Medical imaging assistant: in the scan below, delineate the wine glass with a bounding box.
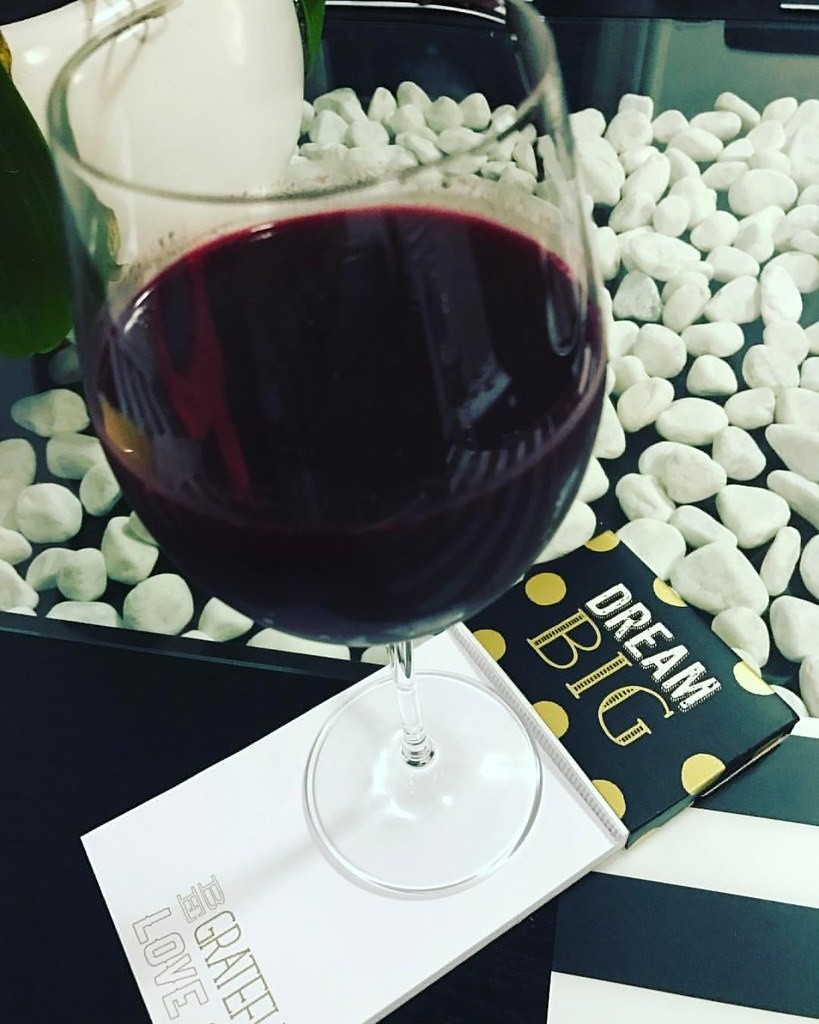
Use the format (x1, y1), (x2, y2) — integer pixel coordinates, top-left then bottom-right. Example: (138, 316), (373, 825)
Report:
(42, 0), (609, 901)
